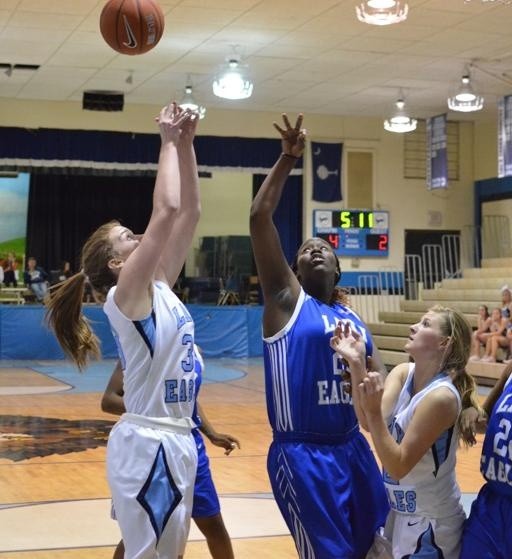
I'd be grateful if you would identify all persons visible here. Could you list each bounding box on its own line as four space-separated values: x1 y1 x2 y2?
0 251 20 289
25 257 48 299
54 261 72 285
42 99 201 559
249 111 391 559
471 287 512 364
101 329 243 559
459 357 512 559
330 303 486 559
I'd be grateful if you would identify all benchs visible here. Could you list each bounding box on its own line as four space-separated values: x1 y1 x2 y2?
364 256 512 386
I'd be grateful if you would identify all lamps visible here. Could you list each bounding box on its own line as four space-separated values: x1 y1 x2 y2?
382 67 485 133
175 85 205 120
355 1 409 26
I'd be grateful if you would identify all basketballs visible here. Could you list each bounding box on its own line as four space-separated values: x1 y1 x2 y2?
99 0 164 55
